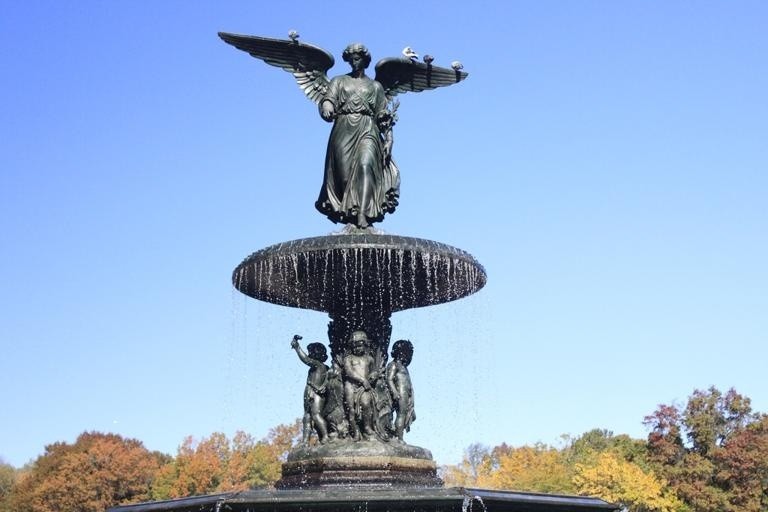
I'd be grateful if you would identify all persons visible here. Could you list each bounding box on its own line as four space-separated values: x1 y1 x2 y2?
341 329 378 442
315 44 400 236
386 338 415 446
290 338 328 446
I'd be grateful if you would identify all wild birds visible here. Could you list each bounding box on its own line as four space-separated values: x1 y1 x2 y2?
451 61 463 71
402 46 419 60
289 30 299 40
423 55 434 64
